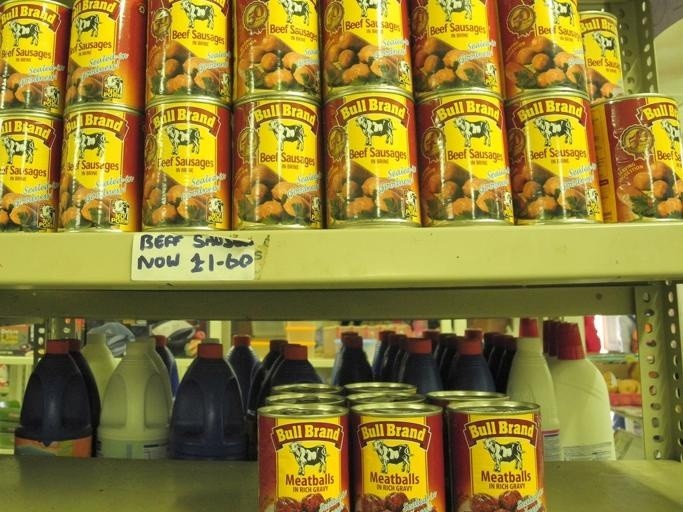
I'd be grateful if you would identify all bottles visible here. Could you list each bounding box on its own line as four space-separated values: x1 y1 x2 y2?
226 334 260 389
15 339 101 459
100 336 177 461
168 343 249 460
81 333 118 391
250 339 324 460
337 320 617 461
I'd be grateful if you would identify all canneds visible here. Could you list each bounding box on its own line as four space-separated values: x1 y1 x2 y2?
0 0 683 233
258 382 546 512
